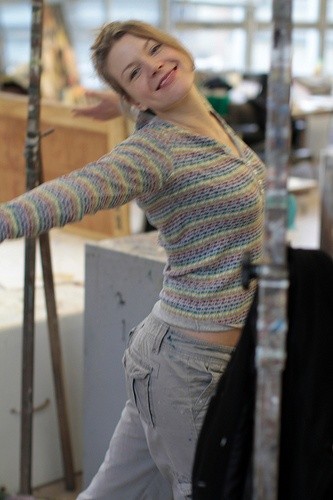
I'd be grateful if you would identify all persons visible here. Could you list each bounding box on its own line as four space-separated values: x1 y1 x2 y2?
1 20 268 500
